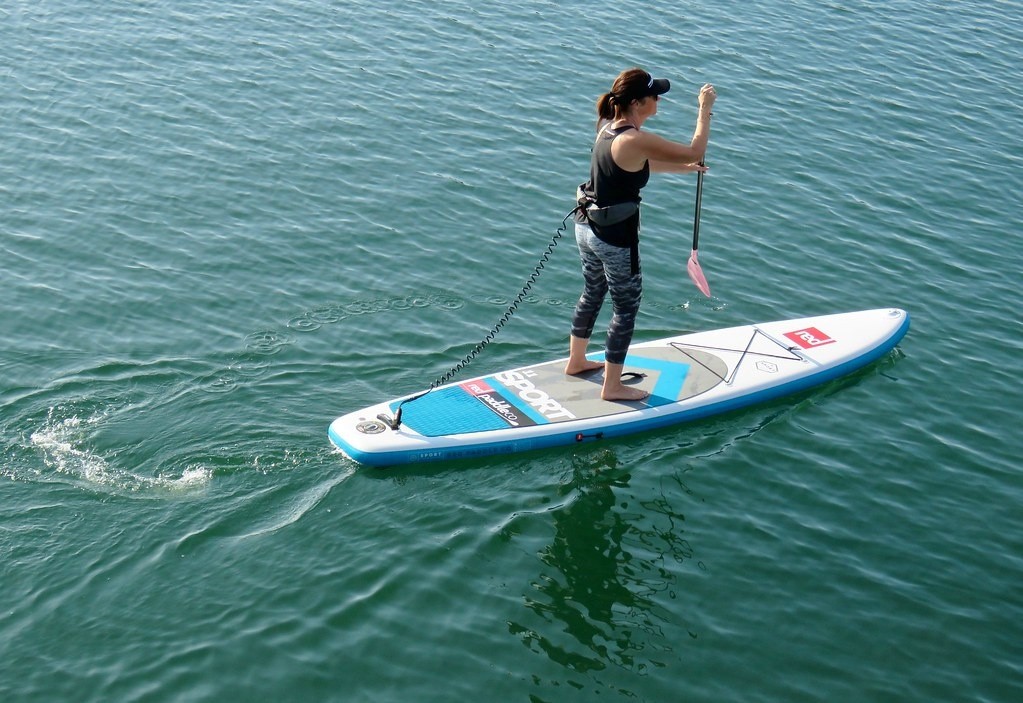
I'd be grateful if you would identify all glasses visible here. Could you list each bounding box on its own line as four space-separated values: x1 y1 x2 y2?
647 94 658 101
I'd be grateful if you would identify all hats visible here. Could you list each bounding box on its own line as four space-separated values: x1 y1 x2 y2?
615 71 670 105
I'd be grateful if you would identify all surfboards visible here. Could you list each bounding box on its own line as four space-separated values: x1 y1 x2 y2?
329 308 909 467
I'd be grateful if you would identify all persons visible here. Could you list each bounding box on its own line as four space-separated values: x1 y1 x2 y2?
565 68 717 401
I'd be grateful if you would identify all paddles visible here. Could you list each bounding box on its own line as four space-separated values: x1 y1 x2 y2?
686 99 714 297
